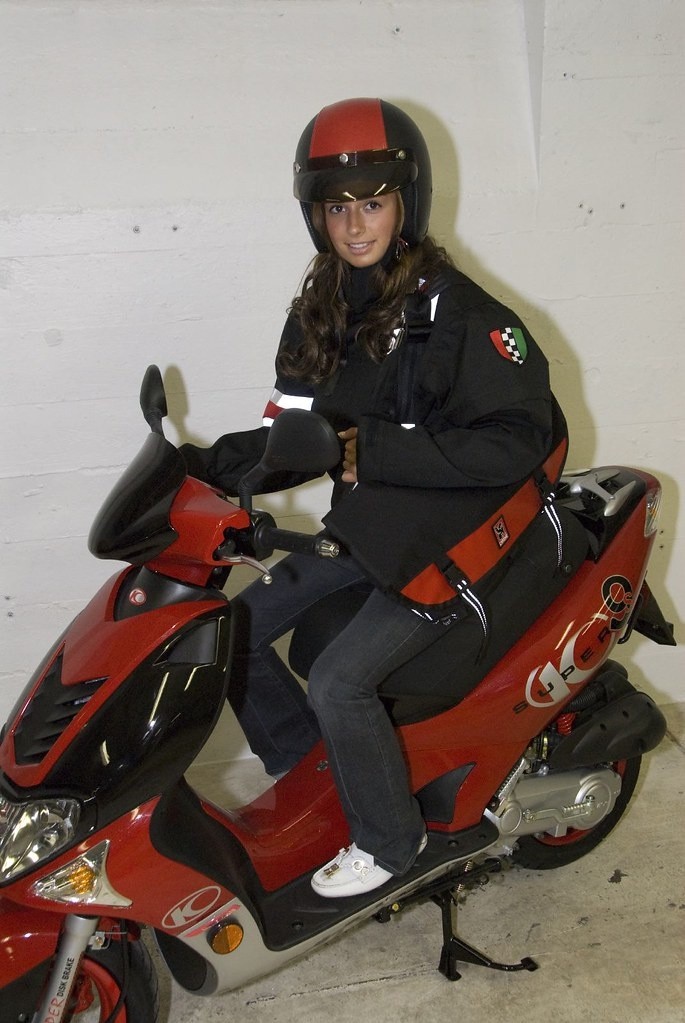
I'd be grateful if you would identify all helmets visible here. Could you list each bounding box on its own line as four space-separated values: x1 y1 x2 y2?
293 97 432 254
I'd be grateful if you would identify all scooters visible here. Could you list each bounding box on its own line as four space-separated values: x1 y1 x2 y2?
0 360 680 1022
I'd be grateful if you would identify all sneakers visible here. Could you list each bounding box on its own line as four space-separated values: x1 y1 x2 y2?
311 833 427 897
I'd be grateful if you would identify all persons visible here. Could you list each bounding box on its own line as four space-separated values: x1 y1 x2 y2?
177 98 554 897
